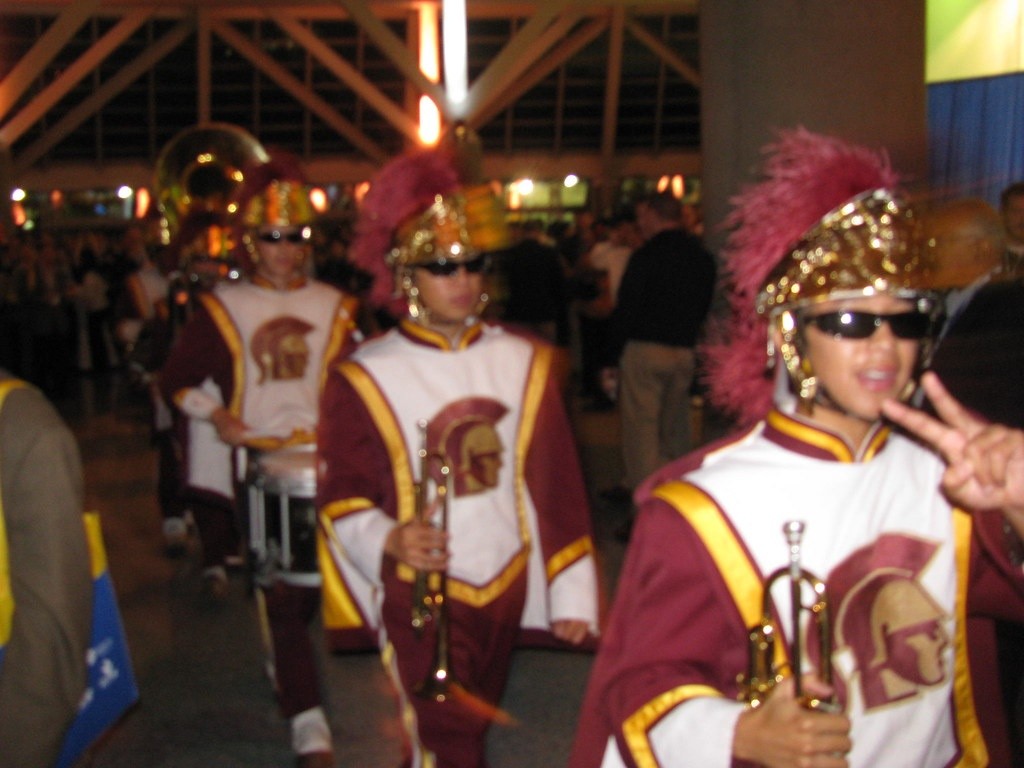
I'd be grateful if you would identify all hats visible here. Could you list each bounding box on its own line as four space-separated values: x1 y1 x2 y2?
174 214 243 261
241 152 326 230
702 128 1004 420
349 122 513 306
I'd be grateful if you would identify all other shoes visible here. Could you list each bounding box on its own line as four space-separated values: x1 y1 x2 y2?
164 518 187 554
616 516 634 542
291 706 332 755
225 556 248 577
202 565 227 598
599 485 632 504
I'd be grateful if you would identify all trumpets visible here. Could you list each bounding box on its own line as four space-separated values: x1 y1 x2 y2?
741 517 852 768
407 420 470 704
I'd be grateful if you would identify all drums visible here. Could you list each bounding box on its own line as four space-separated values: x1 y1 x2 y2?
247 445 327 589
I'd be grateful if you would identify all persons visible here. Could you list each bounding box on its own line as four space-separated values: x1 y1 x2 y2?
600 188 716 543
0 125 1023 768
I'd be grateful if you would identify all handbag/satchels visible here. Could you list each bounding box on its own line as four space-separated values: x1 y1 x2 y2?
0 376 139 768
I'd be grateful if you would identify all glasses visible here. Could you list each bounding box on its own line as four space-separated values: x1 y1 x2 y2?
409 252 492 274
257 231 307 245
797 311 933 340
